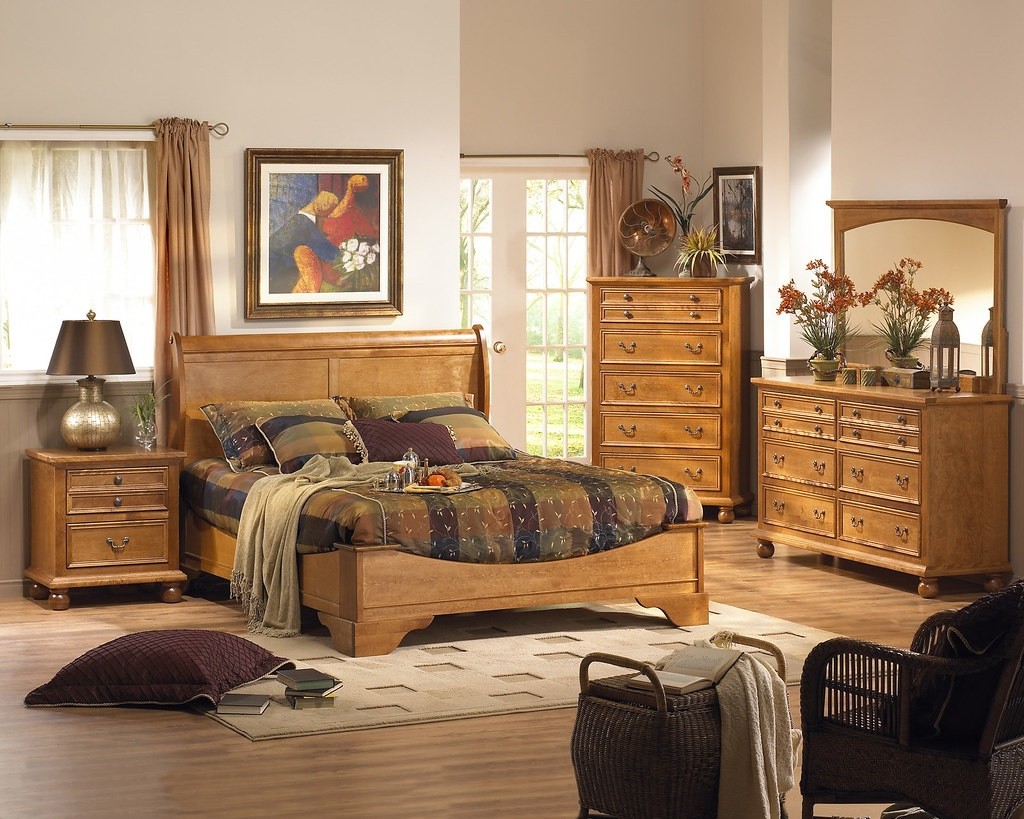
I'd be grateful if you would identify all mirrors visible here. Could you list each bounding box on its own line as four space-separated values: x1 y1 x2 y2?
825 198 1012 406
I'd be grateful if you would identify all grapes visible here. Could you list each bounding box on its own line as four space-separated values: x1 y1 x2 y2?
418 471 439 486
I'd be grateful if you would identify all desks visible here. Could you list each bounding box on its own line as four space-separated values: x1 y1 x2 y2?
570 631 787 819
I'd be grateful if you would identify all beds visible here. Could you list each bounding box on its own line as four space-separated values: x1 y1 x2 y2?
169 332 711 658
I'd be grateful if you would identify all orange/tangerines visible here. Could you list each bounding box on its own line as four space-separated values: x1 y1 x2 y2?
428 475 446 487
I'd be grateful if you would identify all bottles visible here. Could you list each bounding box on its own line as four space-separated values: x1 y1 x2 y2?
402 448 419 462
385 463 423 491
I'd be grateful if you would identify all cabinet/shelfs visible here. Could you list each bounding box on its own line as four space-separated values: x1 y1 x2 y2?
585 276 755 524
749 376 1013 599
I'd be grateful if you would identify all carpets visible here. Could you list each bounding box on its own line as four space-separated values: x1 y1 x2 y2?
205 597 921 742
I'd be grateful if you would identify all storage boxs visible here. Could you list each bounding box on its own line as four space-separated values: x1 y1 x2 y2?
882 369 931 389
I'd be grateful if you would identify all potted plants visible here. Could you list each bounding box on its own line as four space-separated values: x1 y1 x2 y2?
123 380 173 450
671 217 733 278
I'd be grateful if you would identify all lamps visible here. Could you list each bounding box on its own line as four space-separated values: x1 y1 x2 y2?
45 309 136 453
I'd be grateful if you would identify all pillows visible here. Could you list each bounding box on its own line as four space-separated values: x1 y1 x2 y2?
349 391 474 418
255 414 362 473
198 395 347 474
23 630 296 715
390 406 519 465
342 418 464 467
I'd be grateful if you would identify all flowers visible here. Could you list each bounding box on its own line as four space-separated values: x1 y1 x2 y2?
648 154 712 231
775 258 863 351
864 256 954 356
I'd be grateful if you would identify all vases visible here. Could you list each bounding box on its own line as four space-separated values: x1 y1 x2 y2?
809 352 840 380
889 355 919 368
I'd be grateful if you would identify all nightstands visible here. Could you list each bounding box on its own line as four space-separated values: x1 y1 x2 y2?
25 449 188 609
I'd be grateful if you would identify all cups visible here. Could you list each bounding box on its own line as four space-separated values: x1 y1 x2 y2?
842 368 857 384
860 370 877 386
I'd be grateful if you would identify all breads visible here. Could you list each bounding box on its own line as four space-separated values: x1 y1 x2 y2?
436 469 463 486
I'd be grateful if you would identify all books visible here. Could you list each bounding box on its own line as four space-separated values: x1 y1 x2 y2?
274 667 344 711
215 693 272 716
624 646 745 696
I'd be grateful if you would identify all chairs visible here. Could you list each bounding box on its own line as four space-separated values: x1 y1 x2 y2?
798 610 1024 819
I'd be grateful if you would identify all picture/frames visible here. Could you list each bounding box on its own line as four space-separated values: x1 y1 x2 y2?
246 147 406 320
712 165 761 265
